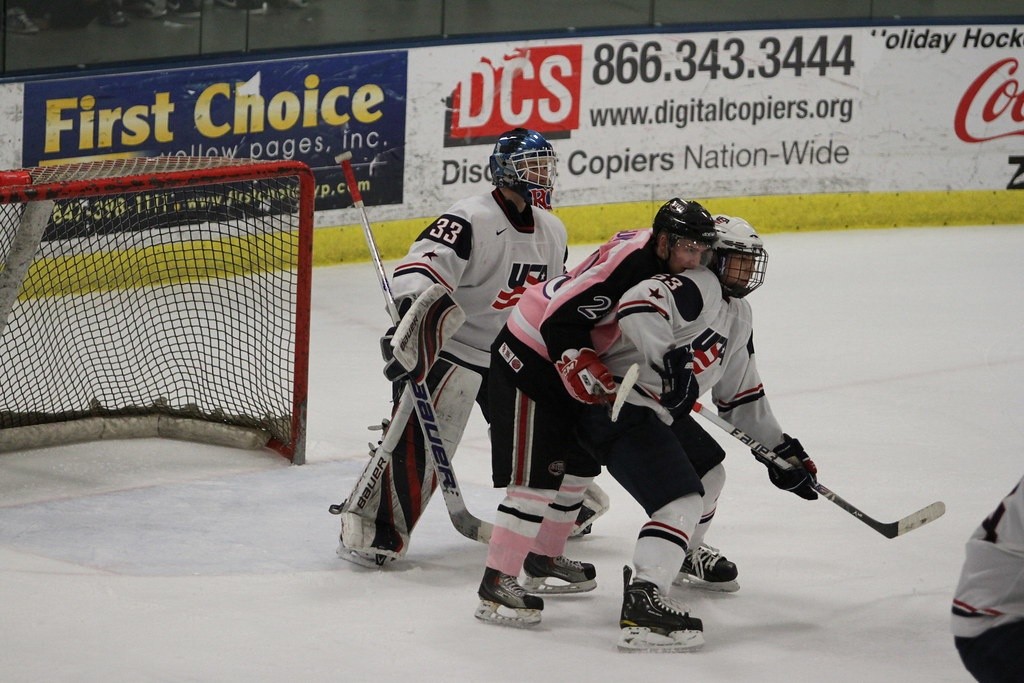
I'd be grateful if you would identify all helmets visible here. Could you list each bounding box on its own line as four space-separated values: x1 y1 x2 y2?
711 213 768 298
490 126 559 209
652 198 719 248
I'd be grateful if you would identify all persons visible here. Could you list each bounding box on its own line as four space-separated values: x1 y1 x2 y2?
329 127 609 569
475 199 720 629
950 476 1024 683
598 214 817 653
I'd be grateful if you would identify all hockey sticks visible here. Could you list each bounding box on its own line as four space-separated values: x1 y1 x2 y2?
331 149 496 545
593 362 641 424
663 385 948 540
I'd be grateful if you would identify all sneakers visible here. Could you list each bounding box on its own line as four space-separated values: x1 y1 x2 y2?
475 567 544 626
616 565 704 649
672 546 740 592
522 551 598 593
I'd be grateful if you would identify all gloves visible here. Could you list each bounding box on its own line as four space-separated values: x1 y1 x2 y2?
553 346 618 404
660 344 699 419
752 432 817 499
